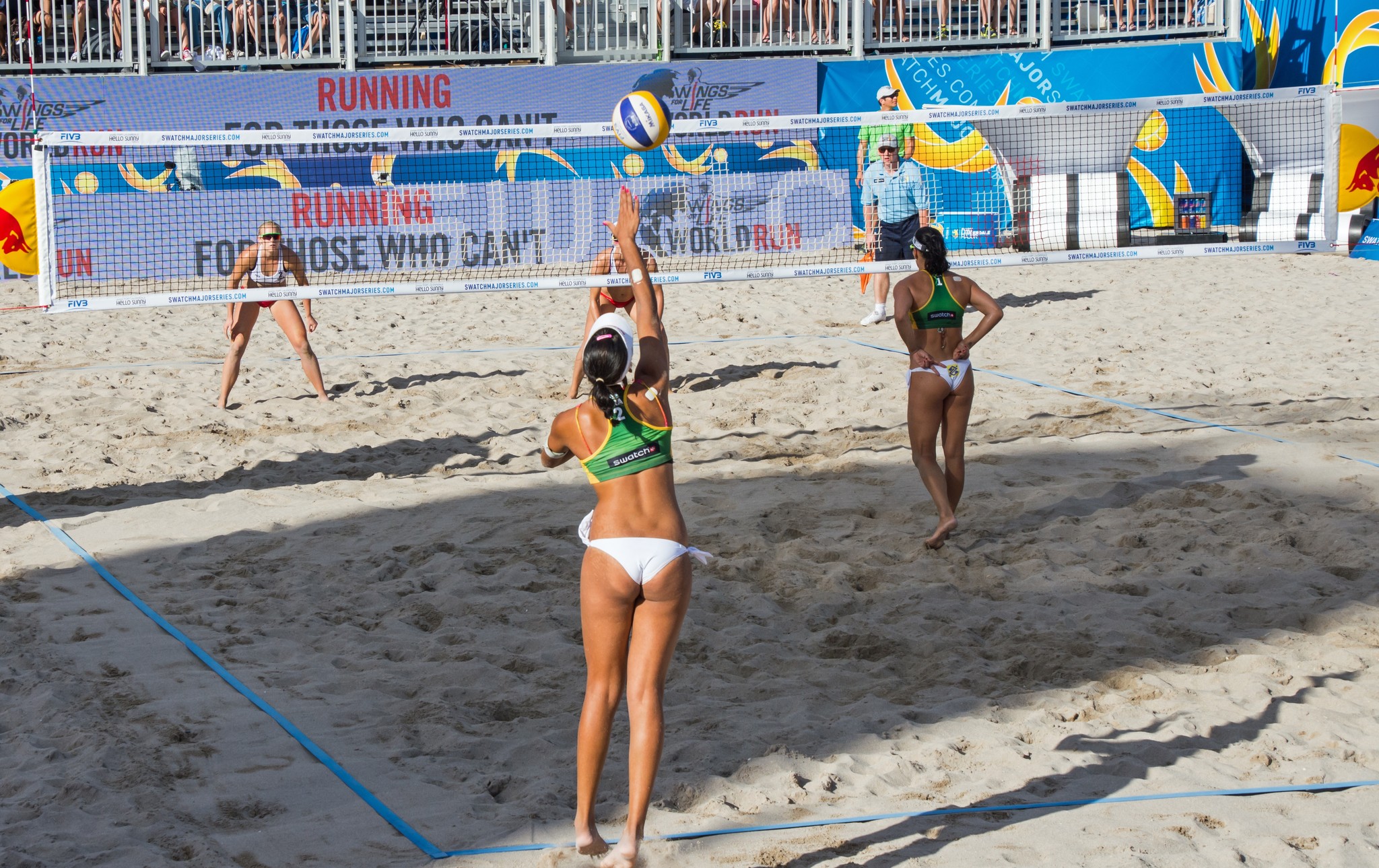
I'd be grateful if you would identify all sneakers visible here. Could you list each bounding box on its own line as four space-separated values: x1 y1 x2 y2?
70 51 81 60
565 25 583 49
115 50 123 59
981 23 997 37
936 24 949 39
160 47 193 62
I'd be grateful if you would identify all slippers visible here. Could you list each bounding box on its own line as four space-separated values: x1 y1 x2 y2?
762 29 795 44
811 37 836 45
1182 20 1203 26
1119 25 1136 31
1145 22 1156 28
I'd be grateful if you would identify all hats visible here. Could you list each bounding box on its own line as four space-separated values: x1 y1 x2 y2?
908 226 933 252
876 85 900 100
582 313 633 386
877 133 898 147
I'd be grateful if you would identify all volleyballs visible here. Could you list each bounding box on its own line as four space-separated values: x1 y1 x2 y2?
612 90 672 152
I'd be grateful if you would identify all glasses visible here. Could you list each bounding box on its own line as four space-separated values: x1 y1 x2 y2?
878 147 895 153
881 93 899 99
910 244 929 252
258 233 281 240
611 233 619 242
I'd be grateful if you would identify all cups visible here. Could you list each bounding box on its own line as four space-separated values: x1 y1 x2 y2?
61 5 72 19
869 50 880 55
752 23 759 31
147 65 155 74
121 67 133 75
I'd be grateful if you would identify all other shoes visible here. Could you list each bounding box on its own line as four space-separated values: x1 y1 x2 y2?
713 19 727 34
684 39 707 46
702 17 716 34
281 50 311 71
231 46 245 57
255 50 266 56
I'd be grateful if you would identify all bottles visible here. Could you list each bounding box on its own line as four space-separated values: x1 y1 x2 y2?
37 36 43 55
175 51 197 58
236 64 261 72
655 39 662 61
670 34 674 46
1163 13 1170 26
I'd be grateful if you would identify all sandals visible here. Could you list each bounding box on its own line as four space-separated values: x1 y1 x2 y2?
0 54 7 61
1009 26 1017 35
992 26 1002 36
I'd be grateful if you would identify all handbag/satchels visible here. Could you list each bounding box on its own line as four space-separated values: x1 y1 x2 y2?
291 24 316 56
1074 2 1112 31
81 27 117 60
451 20 490 51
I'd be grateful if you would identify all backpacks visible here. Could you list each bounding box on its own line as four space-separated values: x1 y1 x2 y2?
708 28 741 60
237 29 267 57
5 16 43 64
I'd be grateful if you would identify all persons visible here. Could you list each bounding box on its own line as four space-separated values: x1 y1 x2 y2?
892 227 1004 549
855 85 915 233
0 0 1203 62
217 221 330 409
860 134 929 326
564 222 675 399
542 186 693 868
